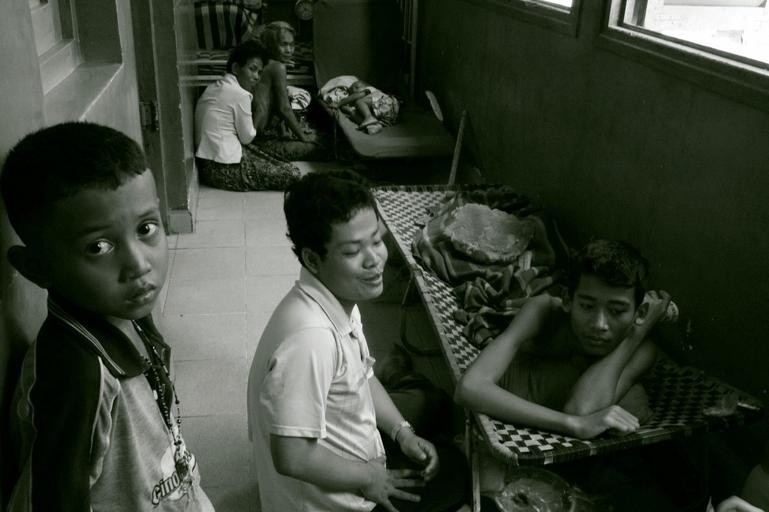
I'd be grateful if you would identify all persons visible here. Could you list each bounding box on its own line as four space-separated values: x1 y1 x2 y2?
452 228 673 441
248 163 472 510
329 80 399 135
192 37 301 191
1 118 214 512
252 20 335 162
715 495 769 512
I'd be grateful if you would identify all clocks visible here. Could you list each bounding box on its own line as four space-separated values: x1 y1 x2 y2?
294 0 313 20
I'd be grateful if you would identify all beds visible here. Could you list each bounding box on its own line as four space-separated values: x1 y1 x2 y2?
370 185 767 468
197 41 313 87
318 94 455 179
195 0 268 49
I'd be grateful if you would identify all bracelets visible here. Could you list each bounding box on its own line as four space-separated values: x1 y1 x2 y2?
391 421 415 444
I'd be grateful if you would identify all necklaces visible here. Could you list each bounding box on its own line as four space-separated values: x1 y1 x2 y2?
122 319 191 480
